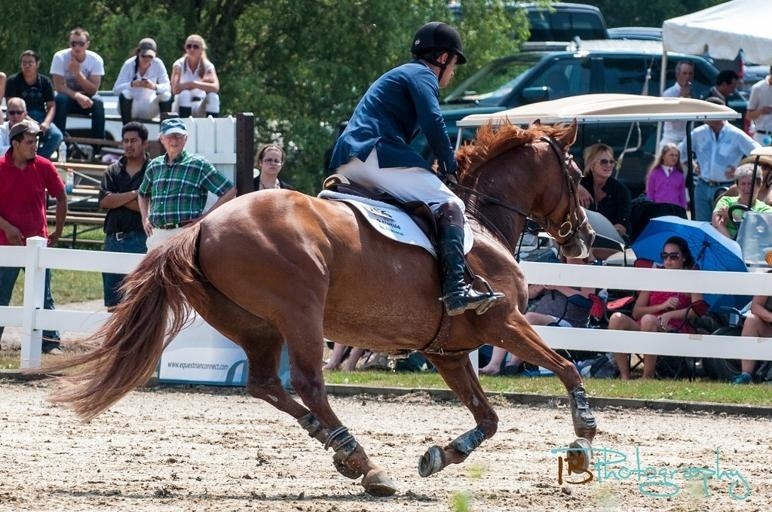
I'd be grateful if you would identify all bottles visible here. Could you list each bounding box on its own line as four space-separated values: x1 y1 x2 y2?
63 167 75 195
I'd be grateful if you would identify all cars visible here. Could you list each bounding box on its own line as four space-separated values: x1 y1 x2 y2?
506 2 662 42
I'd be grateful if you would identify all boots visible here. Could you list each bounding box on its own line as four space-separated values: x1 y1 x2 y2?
119 94 132 124
159 100 173 114
434 201 506 316
181 106 218 118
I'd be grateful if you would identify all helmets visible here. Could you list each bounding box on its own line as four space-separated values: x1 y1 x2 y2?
411 22 467 65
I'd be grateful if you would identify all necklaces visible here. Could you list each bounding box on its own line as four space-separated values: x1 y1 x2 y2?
260 180 278 189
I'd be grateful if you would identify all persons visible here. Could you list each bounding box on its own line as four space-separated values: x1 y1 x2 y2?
171 34 220 118
253 144 299 194
1 120 68 355
140 118 237 257
0 70 7 124
100 123 151 313
328 22 506 318
111 36 172 124
0 97 40 157
323 59 770 385
6 47 64 159
48 27 105 161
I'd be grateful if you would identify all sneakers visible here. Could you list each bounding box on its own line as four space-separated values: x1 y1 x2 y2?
732 372 752 386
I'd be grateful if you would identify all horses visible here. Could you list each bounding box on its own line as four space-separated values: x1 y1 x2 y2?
18 118 596 496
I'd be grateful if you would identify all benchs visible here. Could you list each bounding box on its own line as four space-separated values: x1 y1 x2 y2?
0 107 166 248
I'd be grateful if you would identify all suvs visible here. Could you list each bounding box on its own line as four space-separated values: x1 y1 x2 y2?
412 49 749 199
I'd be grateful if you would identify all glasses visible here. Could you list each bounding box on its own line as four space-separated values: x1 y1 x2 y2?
262 157 282 164
8 110 24 114
24 61 35 66
71 40 85 46
597 159 615 165
661 250 680 261
186 44 199 48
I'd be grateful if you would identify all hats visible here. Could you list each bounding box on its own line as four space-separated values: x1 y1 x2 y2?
139 38 157 57
8 121 44 137
160 118 188 137
740 155 772 167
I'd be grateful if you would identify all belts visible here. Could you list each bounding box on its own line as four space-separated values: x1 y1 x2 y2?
700 175 734 186
113 233 123 241
757 129 772 134
157 222 193 228
